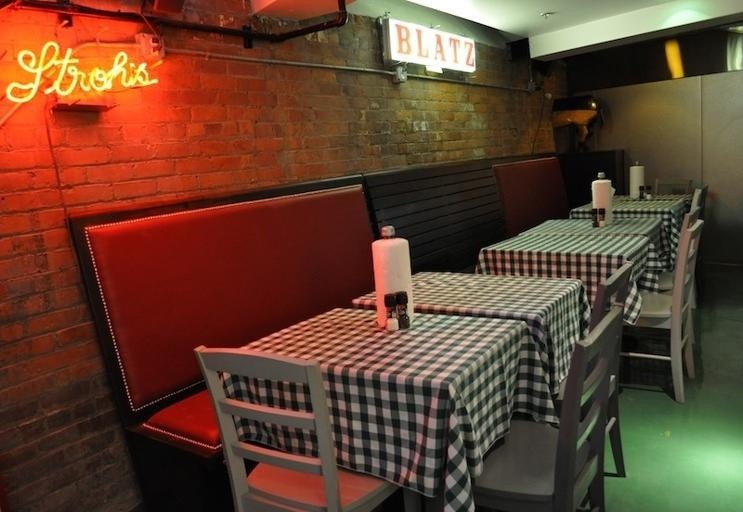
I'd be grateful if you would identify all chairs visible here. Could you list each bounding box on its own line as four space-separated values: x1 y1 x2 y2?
475 303 628 511
622 218 705 403
551 254 635 508
657 209 703 310
692 181 709 211
194 341 423 510
656 175 694 195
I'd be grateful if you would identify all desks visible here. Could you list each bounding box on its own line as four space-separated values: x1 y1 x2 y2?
214 299 563 512
352 268 595 424
475 213 663 327
568 191 693 267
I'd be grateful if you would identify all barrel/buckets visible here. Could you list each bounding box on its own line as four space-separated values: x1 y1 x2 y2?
591 178 616 225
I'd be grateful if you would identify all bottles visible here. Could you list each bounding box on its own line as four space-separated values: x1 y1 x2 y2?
591 172 614 227
371 226 415 332
629 160 652 200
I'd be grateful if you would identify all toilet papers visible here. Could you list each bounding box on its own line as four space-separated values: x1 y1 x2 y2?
372 237 414 326
629 165 645 200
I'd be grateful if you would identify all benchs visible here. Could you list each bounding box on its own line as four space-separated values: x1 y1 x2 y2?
68 174 389 511
493 152 569 233
